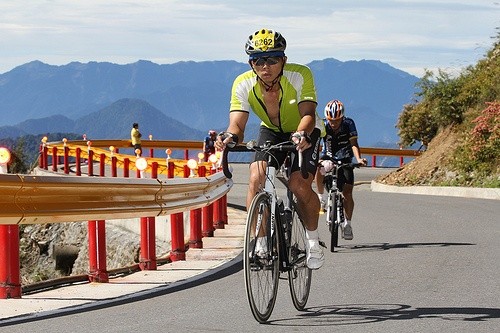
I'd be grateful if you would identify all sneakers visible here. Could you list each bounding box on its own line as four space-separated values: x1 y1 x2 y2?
249 246 268 259
306 243 325 269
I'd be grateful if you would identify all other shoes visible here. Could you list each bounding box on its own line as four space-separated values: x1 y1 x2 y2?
344 224 353 240
319 199 326 211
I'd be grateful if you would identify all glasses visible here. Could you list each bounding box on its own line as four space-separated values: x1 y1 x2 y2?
252 57 280 66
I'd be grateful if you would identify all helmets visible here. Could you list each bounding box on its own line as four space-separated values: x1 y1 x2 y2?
244 28 287 59
324 99 344 120
208 130 216 135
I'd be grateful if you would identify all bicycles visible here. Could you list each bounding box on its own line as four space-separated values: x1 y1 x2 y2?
219 134 328 325
318 159 369 253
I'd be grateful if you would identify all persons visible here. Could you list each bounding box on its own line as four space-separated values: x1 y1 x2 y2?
318 100 368 240
131 123 142 157
203 131 218 162
213 28 327 270
